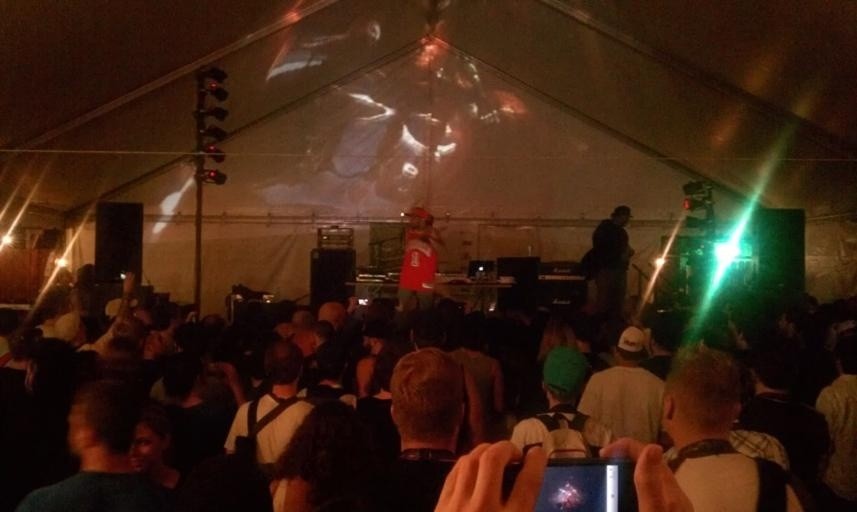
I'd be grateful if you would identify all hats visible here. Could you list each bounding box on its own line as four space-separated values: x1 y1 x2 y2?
103 298 137 318
610 205 633 217
542 345 589 396
54 311 80 342
404 207 429 220
609 323 649 362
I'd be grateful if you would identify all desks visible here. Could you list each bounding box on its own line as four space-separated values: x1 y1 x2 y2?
342 280 513 312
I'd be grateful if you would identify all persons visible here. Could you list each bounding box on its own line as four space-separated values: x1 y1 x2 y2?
590 204 638 312
1 257 856 510
399 203 444 309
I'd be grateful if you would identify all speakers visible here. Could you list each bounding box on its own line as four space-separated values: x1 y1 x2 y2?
94 201 142 282
310 245 356 305
496 256 540 314
754 207 804 288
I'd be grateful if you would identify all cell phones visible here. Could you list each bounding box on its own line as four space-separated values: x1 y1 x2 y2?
503 458 638 512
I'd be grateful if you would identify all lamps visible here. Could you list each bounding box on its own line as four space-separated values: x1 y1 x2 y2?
682 181 717 266
193 68 231 187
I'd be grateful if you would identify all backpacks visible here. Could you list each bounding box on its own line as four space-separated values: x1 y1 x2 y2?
533 411 593 460
226 395 298 511
